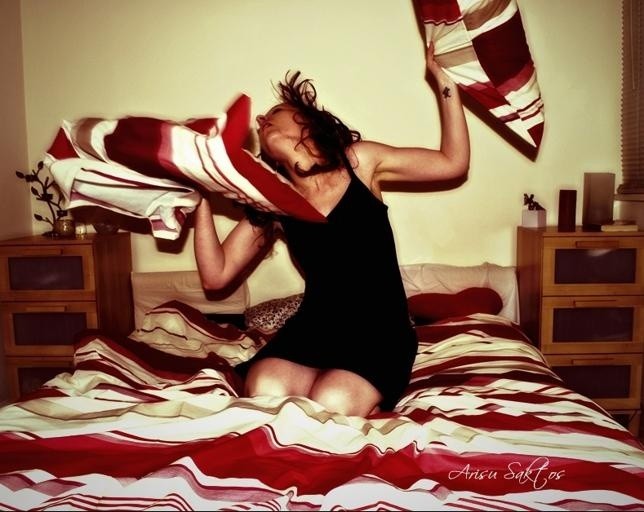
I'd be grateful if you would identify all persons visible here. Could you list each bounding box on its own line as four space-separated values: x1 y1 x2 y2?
193 39 472 420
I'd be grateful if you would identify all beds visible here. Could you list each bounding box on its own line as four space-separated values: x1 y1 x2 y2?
0 321 644 510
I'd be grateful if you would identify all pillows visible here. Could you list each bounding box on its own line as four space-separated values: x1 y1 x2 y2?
400 262 523 329
413 0 547 154
127 270 252 329
40 93 324 245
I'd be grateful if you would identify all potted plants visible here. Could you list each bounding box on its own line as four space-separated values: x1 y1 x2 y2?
521 193 547 228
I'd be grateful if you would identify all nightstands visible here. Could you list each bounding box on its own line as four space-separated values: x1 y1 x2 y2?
0 231 135 403
515 225 643 428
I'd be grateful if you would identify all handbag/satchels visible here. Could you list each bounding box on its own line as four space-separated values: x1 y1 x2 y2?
244 293 305 335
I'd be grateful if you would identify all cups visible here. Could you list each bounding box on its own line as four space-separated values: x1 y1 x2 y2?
558 188 577 233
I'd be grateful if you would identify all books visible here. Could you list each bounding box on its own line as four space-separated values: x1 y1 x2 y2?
600 219 639 232
